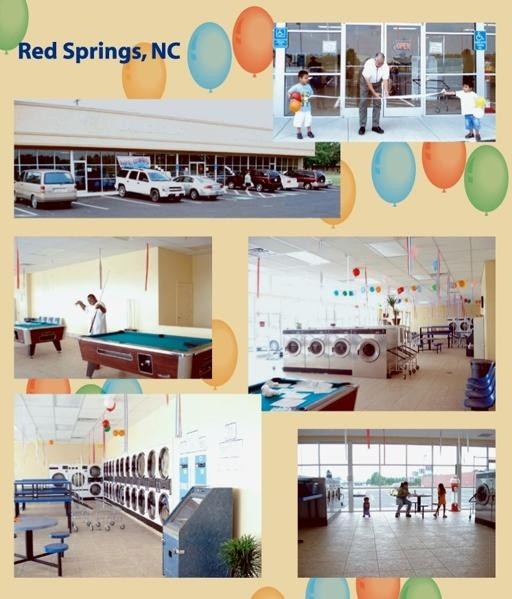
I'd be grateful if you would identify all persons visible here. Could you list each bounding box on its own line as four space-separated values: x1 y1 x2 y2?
285 54 292 68
76 296 107 335
287 71 318 139
307 57 324 90
432 483 447 519
358 54 391 135
442 84 486 142
395 481 412 517
362 497 371 518
243 171 252 193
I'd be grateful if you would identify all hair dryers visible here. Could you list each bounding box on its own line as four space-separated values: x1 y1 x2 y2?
298 478 341 526
104 443 174 527
283 330 305 372
305 330 329 373
353 326 397 379
447 317 473 333
329 330 352 375
475 470 495 530
49 464 104 499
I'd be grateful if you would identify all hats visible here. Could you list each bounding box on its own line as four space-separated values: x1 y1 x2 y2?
172 175 225 201
321 171 333 188
278 174 297 192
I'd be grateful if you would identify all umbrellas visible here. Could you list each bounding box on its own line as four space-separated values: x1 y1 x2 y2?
297 133 303 139
372 126 384 133
476 134 480 140
308 131 314 138
465 133 474 138
359 127 366 135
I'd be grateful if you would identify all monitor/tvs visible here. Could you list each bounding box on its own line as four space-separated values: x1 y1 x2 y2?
252 576 442 599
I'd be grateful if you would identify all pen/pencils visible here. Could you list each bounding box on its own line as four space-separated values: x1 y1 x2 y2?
14 168 79 210
225 169 282 193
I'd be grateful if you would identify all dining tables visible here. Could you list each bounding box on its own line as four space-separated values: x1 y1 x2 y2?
393 310 400 324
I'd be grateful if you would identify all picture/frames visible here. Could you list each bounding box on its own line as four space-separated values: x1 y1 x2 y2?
14 321 65 356
78 330 212 379
248 377 359 411
14 515 61 568
14 478 72 533
409 494 432 512
419 326 453 352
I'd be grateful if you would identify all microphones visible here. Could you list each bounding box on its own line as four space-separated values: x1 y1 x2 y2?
410 502 418 513
419 505 428 519
433 343 442 353
44 543 68 576
48 532 70 558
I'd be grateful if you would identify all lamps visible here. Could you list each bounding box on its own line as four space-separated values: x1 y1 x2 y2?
465 362 495 408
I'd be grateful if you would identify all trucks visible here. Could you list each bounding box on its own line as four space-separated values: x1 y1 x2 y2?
122 6 276 99
326 261 478 322
45 439 55 447
475 97 486 108
325 141 508 232
289 90 302 113
27 378 145 395
98 397 129 438
0 0 28 55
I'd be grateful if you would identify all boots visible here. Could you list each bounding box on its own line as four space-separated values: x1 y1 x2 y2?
113 167 186 203
293 169 326 190
